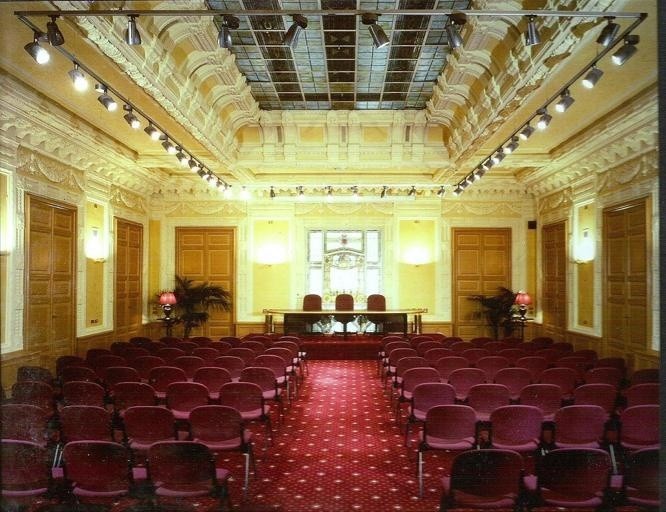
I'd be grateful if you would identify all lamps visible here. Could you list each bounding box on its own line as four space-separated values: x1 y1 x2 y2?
217 15 240 48
281 15 308 46
23 38 233 198
436 34 640 197
515 289 531 320
593 17 621 47
444 13 467 47
47 16 64 46
160 291 177 320
361 14 390 48
126 16 141 46
270 188 276 198
525 15 542 47
408 186 416 196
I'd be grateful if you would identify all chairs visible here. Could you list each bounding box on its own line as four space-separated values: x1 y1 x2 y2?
363 295 388 337
334 294 355 339
301 294 324 336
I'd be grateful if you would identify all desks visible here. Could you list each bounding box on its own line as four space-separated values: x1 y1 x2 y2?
512 317 535 341
263 309 427 332
156 318 179 336
